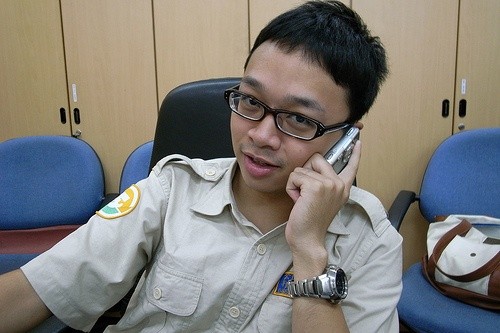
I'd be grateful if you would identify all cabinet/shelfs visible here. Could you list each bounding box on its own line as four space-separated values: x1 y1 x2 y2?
152 0 352 112
0 0 159 194
350 0 500 274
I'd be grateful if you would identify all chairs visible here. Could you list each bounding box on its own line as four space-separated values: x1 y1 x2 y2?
0 134 106 333
384 126 500 333
54 76 243 333
97 140 155 212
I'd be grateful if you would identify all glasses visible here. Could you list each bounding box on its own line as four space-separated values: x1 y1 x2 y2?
225 84 351 141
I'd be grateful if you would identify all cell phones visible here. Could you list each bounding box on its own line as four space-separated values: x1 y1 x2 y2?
323 127 361 175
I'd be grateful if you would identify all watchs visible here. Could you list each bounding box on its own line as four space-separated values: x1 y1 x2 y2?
288 264 348 304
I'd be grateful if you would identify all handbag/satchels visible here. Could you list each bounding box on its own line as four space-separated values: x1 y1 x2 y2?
425 214 500 312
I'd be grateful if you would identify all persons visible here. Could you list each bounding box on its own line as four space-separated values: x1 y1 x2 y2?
0 0 403 333
